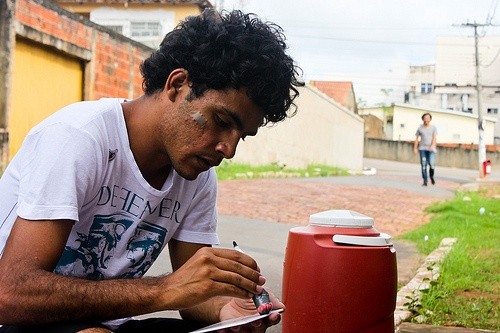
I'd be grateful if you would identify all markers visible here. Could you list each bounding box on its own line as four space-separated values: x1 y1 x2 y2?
232 241 271 316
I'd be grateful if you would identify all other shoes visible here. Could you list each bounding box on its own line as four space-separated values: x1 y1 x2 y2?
430 177 435 184
422 180 427 186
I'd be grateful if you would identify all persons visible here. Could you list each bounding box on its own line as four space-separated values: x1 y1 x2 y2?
1 6 306 333
414 112 437 186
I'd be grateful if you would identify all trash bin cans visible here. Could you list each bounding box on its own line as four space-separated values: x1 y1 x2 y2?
281 209 397 333
483 159 491 175
